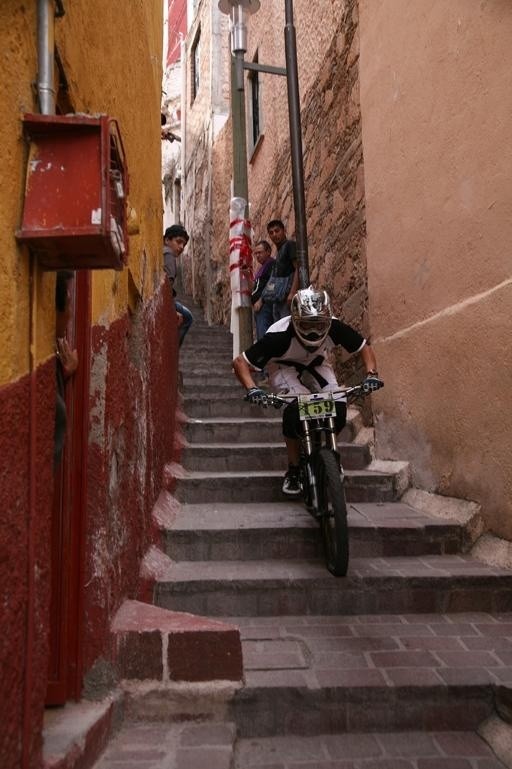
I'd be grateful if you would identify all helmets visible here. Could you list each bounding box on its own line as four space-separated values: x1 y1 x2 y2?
290 283 335 352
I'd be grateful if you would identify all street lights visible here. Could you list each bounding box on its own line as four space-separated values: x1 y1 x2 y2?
217 1 263 357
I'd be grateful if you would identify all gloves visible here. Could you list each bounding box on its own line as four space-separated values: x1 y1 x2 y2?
246 386 269 410
362 368 384 394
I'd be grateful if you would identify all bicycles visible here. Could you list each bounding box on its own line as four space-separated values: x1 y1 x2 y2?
243 378 381 578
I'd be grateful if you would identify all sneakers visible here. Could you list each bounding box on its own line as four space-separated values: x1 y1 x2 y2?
281 461 307 496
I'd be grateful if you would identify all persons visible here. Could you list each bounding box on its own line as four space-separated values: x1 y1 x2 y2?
231 285 381 500
54 270 81 483
266 218 299 321
252 239 275 372
161 224 195 355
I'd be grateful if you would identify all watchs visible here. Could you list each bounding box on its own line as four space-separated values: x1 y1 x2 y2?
367 369 378 377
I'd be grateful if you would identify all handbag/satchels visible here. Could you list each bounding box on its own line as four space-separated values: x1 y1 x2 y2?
260 275 293 306
